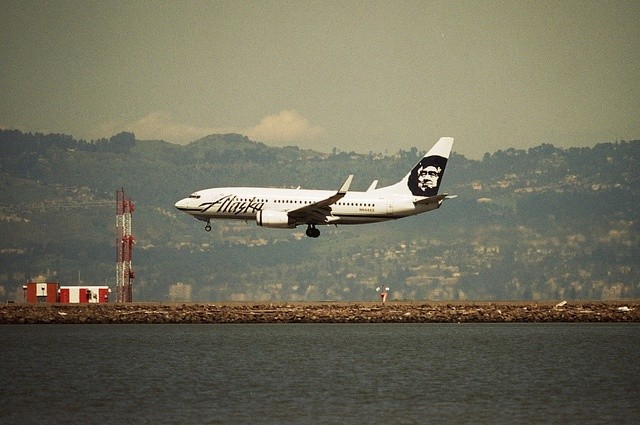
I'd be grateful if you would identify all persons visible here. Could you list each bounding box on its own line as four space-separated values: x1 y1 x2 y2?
416 165 442 192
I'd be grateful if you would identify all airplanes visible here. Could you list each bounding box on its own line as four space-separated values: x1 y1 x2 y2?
175 136 457 238
